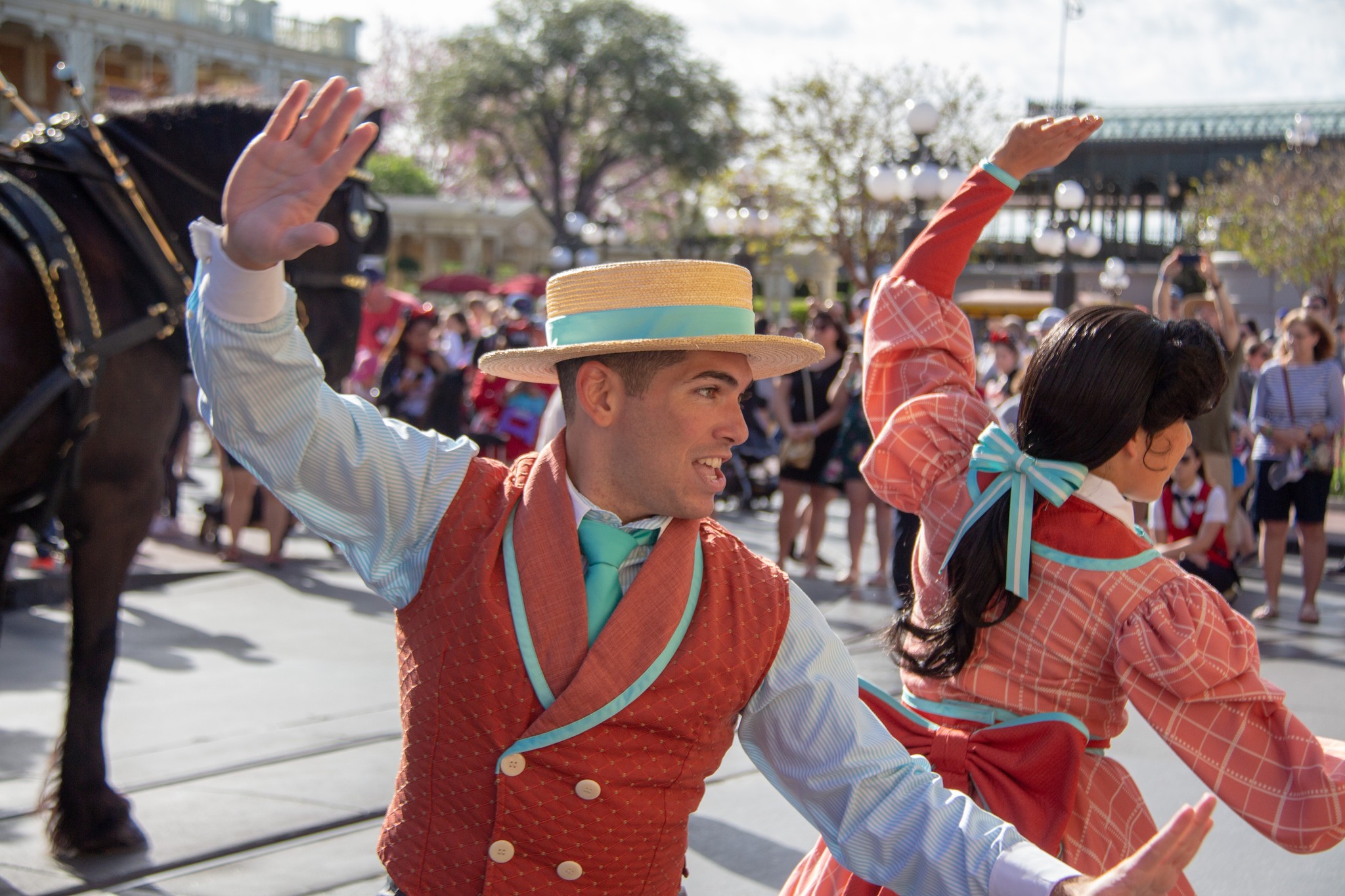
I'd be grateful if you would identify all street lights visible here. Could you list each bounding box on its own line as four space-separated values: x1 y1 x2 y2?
861 101 965 266
1034 182 1100 310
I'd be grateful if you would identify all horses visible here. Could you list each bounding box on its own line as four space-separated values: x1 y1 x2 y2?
1 85 396 871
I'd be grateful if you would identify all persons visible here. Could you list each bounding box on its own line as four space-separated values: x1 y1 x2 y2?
1148 445 1240 603
221 448 289 565
780 113 1345 896
977 309 1068 434
1230 294 1345 624
1152 246 1248 501
769 289 920 606
344 269 558 461
188 75 1219 896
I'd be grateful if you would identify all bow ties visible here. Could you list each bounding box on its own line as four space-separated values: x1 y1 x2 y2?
1172 494 1197 505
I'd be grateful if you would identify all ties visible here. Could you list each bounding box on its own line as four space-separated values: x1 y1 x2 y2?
578 515 660 649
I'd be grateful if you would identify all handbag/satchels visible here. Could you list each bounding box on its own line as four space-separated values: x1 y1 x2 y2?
1268 446 1304 490
780 436 814 469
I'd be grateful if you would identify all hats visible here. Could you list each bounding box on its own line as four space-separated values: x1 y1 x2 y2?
479 259 825 385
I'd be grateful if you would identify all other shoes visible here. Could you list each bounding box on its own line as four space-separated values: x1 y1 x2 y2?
1299 601 1318 624
1252 604 1278 620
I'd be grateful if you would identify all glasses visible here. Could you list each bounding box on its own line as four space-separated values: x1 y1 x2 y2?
1179 455 1190 464
